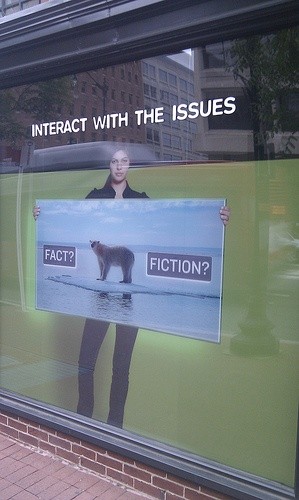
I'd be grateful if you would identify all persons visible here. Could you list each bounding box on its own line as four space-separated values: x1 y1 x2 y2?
32 146 230 429
